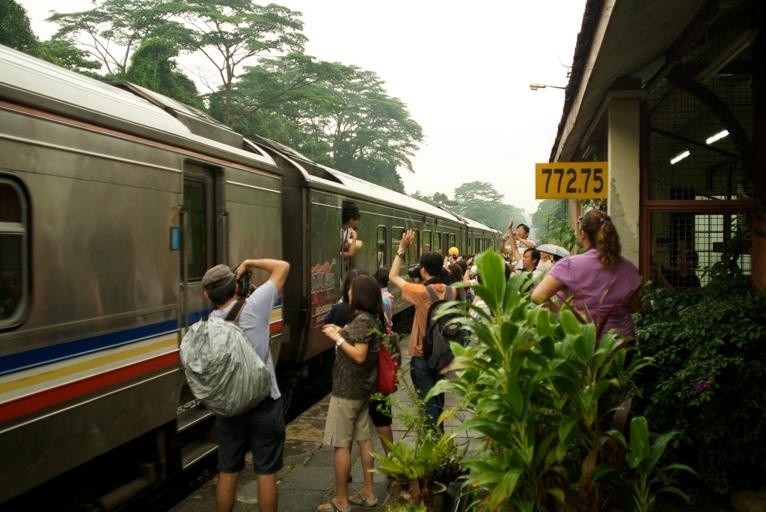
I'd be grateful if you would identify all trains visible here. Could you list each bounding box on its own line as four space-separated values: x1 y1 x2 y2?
0 42 505 512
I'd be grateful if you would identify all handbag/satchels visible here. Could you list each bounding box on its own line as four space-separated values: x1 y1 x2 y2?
377 326 400 394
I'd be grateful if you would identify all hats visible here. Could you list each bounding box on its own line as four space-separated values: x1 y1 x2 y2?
202 264 236 293
448 247 459 257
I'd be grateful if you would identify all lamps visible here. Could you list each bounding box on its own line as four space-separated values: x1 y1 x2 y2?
705 128 730 146
529 83 566 91
669 146 692 166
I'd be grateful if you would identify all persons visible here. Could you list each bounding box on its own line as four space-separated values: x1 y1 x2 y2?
462 208 642 477
654 246 700 296
201 257 290 511
388 229 469 435
319 206 403 511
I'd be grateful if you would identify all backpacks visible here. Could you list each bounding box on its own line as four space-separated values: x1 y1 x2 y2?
418 284 465 373
179 299 271 418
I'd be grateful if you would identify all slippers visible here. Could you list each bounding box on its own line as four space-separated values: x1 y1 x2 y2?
347 490 379 507
318 497 353 512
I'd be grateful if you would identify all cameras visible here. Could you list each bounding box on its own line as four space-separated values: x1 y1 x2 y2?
233 269 250 296
